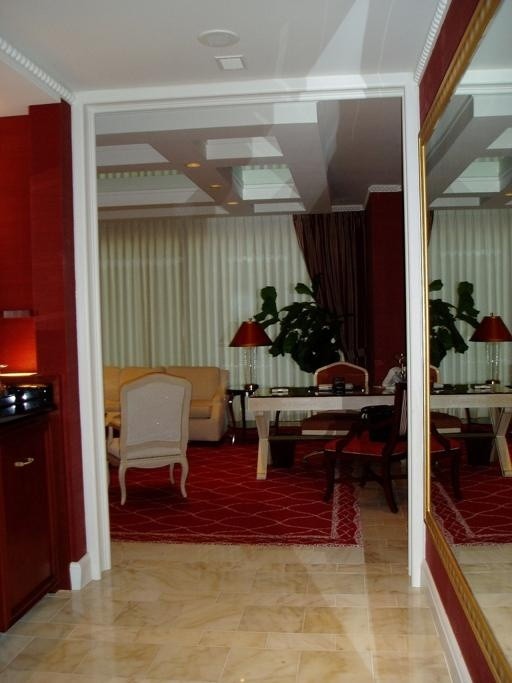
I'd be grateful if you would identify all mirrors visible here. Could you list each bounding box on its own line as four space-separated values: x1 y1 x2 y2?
417 0 512 683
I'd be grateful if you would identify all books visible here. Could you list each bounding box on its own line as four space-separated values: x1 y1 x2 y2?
270 387 308 397
470 381 492 390
318 381 333 390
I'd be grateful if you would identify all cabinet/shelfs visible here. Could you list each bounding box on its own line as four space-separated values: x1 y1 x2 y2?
0 401 63 632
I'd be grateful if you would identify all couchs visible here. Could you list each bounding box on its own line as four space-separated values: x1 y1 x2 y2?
104 367 228 446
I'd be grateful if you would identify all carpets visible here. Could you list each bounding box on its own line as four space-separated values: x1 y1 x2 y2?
107 426 362 548
431 438 512 546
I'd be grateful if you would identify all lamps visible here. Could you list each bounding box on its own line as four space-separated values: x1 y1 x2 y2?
468 313 512 384
229 318 273 390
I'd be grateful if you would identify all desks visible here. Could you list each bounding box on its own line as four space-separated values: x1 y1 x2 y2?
248 386 407 481
429 383 512 478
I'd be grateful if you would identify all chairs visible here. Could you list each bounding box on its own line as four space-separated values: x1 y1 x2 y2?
302 360 407 514
106 372 193 506
430 365 468 500
